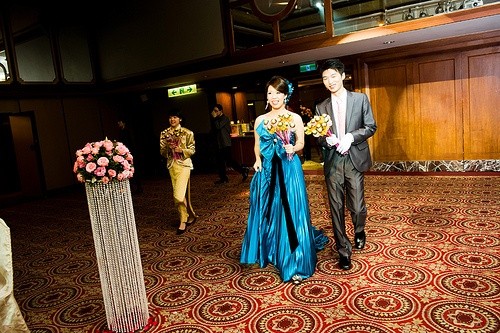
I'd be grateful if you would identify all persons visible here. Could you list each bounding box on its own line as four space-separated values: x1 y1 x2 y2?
211 104 250 183
239 75 328 284
316 59 377 270
160 109 199 234
116 116 143 193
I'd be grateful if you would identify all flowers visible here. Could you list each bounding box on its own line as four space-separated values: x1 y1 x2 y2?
160 126 188 161
263 111 297 162
303 113 349 156
73 136 134 188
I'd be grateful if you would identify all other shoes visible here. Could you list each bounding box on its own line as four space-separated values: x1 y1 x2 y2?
242 166 249 181
186 217 195 225
177 224 185 234
215 179 228 184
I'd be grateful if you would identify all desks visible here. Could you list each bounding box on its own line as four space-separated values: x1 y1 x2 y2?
231 134 257 166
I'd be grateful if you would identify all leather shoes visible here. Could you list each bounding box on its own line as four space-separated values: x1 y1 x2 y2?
355 230 365 249
340 254 350 270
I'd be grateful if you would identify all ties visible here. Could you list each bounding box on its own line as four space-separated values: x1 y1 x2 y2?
336 100 344 142
